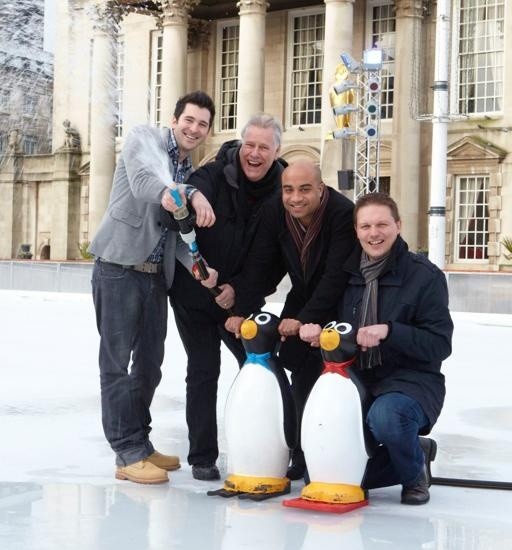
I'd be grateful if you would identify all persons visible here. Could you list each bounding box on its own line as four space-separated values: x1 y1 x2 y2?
159 111 291 482
86 89 216 486
223 155 360 480
297 191 455 503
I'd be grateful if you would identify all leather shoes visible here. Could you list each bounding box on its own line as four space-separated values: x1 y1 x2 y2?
401 436 436 505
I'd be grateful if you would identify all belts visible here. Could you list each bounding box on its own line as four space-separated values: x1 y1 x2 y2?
123 262 157 274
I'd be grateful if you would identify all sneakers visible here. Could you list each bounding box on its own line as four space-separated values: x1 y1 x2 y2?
115 449 179 485
193 463 221 480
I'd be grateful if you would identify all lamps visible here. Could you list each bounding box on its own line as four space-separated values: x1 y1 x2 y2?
332 52 381 144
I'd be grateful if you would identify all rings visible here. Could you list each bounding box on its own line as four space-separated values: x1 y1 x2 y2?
224 303 228 307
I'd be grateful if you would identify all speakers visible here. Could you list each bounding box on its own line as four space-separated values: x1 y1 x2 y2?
337 169 354 190
